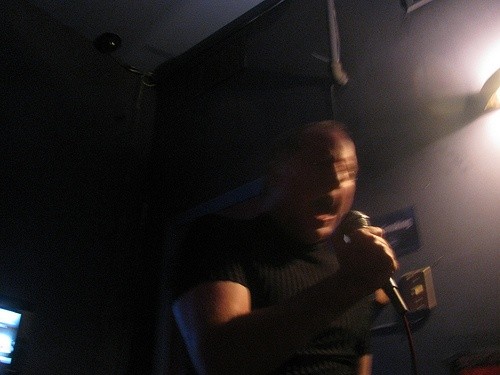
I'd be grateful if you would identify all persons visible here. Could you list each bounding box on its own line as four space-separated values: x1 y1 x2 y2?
165 119 397 375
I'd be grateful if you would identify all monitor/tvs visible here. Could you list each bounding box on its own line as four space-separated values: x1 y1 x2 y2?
0 305 32 370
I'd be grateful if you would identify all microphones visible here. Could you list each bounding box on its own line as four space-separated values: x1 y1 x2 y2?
341 211 409 315
